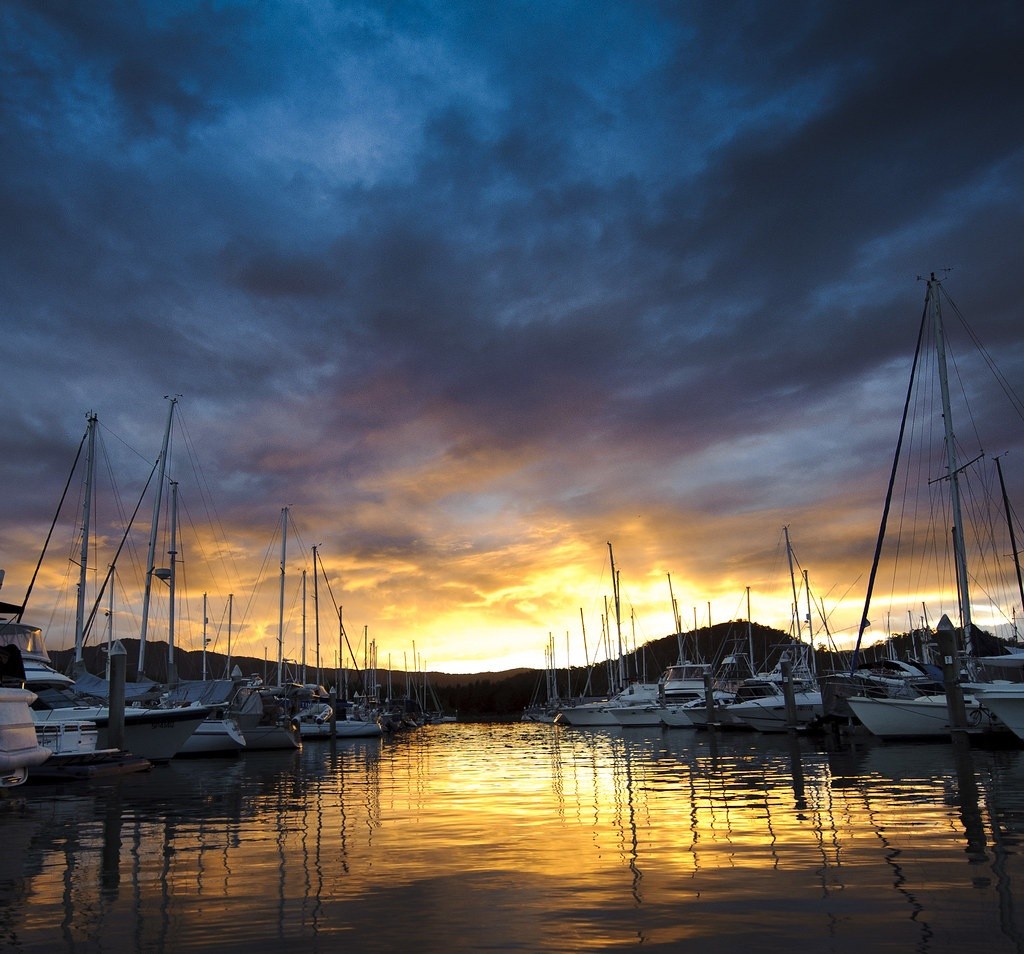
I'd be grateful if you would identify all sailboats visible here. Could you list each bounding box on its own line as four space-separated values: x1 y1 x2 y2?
519 272 1024 747
1 391 459 788
